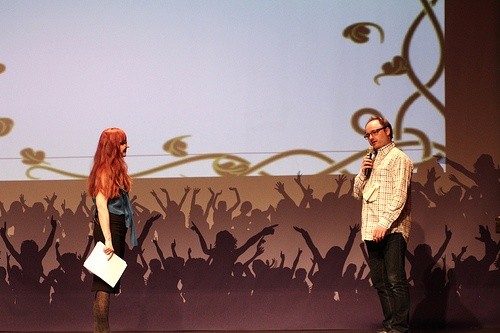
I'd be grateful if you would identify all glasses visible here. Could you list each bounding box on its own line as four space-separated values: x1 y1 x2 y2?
364 127 386 140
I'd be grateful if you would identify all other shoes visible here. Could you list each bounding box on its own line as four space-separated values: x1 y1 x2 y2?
377 327 400 333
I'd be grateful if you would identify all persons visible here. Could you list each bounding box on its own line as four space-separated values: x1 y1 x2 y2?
87 127 132 333
351 116 413 333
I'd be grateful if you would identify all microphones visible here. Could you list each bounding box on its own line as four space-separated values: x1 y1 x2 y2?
365 146 374 177
